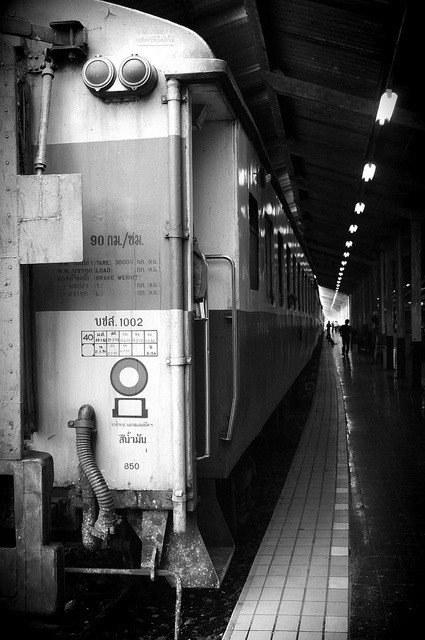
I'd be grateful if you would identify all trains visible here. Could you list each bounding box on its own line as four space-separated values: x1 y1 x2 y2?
0 0 324 640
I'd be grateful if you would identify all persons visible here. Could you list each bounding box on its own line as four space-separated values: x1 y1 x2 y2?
326 320 331 335
339 319 353 356
